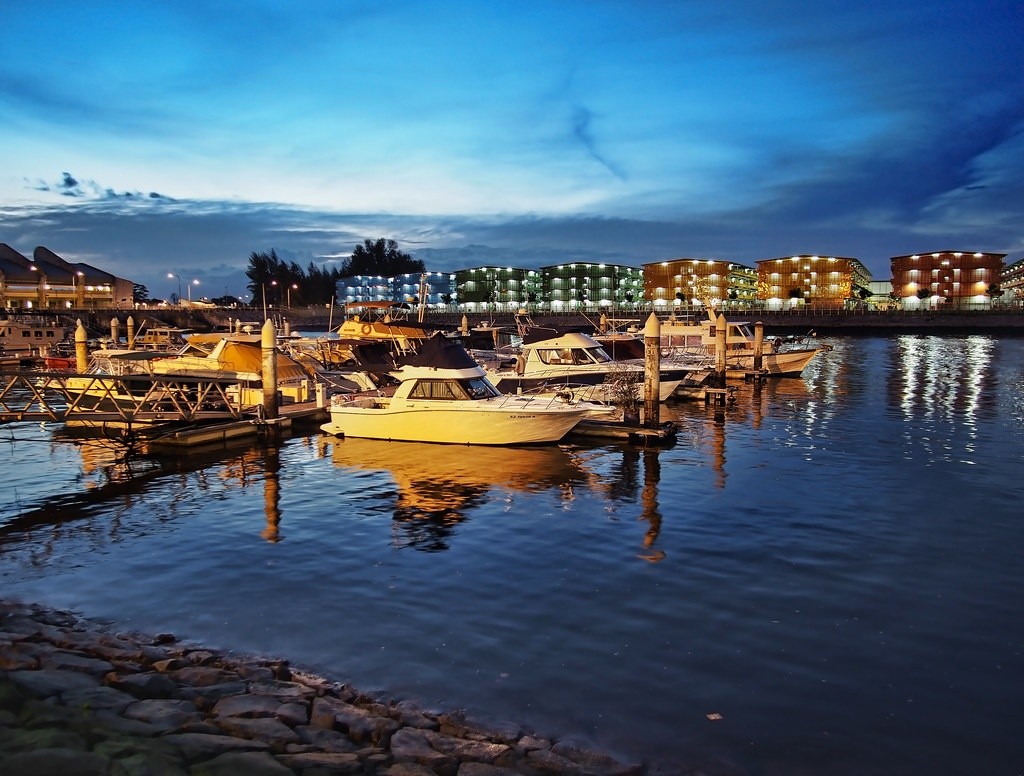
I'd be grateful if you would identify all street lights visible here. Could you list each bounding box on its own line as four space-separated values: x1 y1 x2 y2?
288 284 298 309
272 281 283 306
243 295 249 307
73 271 85 307
238 297 242 307
188 280 199 301
168 273 181 303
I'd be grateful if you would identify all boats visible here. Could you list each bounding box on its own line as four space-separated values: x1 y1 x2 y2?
431 297 819 402
0 314 333 424
726 376 811 401
319 328 592 444
337 274 429 339
319 436 587 512
